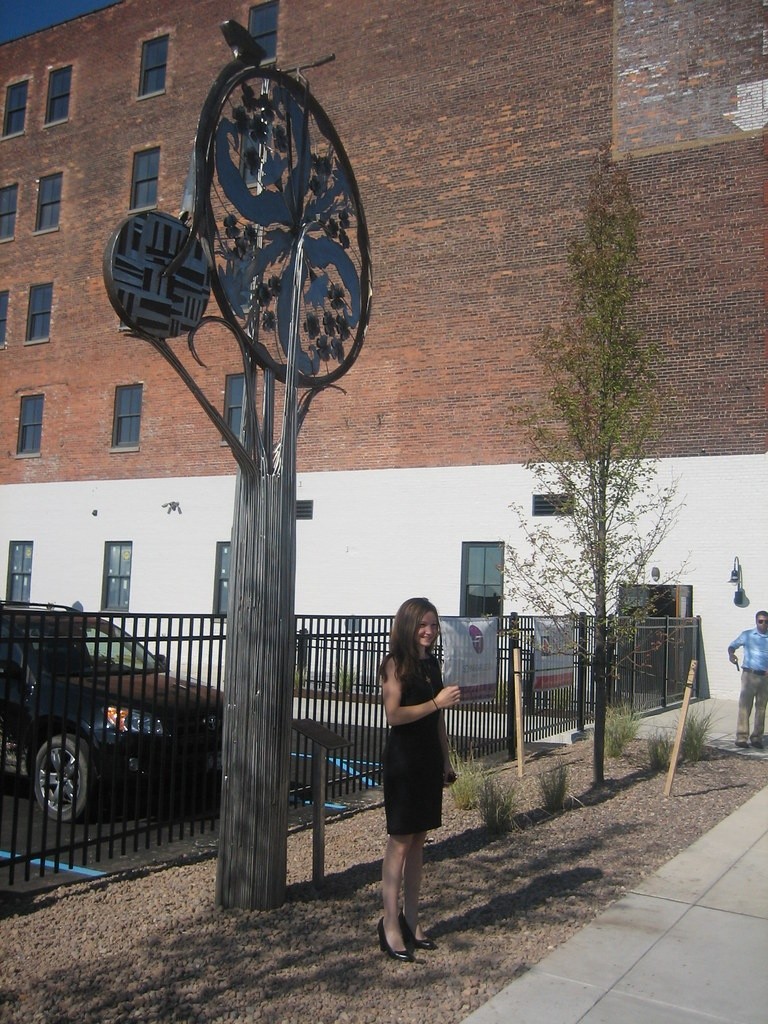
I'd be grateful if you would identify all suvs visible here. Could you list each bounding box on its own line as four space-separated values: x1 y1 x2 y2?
0 599 224 826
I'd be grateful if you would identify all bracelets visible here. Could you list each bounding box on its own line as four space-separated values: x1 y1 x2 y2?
432 699 438 710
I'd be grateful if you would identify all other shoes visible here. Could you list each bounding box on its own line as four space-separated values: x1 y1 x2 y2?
752 740 762 749
735 742 749 748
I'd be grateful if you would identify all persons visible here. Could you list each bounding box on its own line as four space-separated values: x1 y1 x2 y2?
728 611 768 749
377 598 460 962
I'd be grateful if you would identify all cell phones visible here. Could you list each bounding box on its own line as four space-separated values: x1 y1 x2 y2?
736 661 739 671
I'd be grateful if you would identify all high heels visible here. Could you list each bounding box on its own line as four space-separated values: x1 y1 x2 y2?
398 911 438 950
378 915 415 963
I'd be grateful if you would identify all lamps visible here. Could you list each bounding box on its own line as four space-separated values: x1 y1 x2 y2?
729 556 750 607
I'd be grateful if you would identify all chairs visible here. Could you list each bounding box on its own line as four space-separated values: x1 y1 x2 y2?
44 624 94 668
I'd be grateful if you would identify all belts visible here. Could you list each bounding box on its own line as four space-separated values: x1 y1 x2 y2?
743 668 766 675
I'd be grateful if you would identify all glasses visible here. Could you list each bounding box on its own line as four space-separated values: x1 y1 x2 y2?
757 620 768 624
